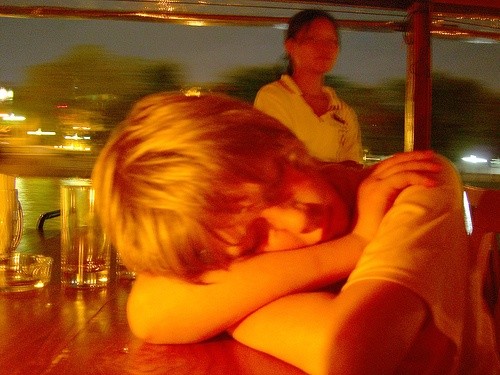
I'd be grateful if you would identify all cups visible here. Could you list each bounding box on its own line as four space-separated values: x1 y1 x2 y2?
60 180 111 290
0 173 23 261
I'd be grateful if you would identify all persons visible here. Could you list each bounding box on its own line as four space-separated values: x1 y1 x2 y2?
88 90 500 375
250 7 365 170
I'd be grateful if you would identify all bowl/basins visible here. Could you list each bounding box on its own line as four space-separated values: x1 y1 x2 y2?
0 252 55 293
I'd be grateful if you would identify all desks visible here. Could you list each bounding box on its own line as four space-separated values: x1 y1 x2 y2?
1 226 310 375
